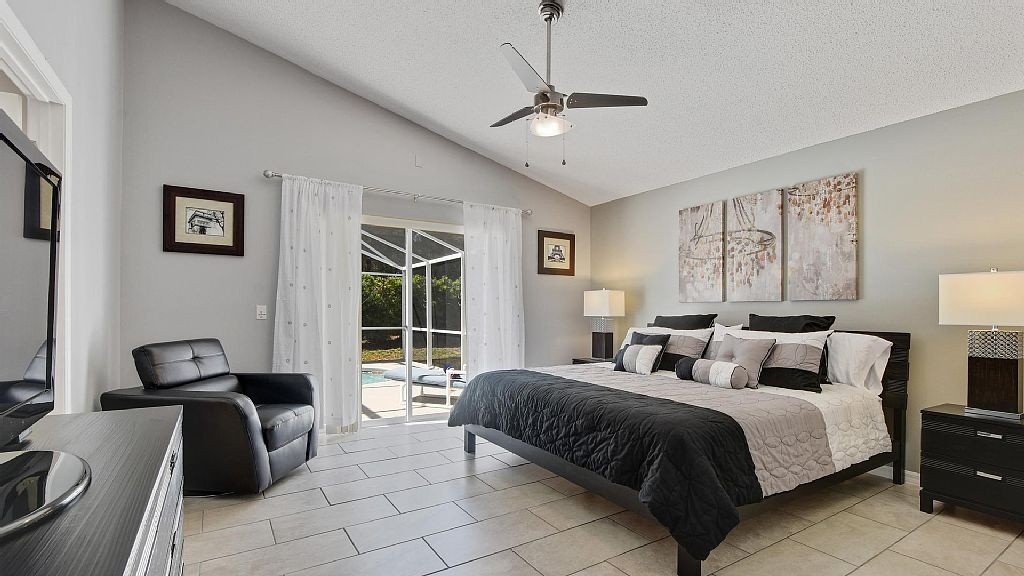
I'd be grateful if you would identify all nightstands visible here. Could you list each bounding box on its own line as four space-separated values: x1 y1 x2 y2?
919 403 1024 524
572 357 613 365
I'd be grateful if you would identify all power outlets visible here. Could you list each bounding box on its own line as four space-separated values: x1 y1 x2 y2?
256 305 270 319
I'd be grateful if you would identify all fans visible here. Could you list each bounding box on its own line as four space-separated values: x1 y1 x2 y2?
490 1 648 128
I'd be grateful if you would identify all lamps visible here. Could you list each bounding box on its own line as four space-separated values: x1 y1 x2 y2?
939 268 1024 419
524 85 572 168
584 287 625 359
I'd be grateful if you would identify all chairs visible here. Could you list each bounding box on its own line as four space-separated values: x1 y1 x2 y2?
383 367 466 409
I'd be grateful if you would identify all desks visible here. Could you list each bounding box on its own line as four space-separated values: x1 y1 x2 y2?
0 405 185 576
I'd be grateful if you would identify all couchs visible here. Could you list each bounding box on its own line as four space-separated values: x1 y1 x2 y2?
100 338 319 495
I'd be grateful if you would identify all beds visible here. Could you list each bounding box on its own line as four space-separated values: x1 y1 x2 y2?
447 330 911 576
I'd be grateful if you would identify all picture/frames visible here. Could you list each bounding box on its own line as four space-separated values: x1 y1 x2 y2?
162 184 244 256
538 230 575 276
23 162 53 240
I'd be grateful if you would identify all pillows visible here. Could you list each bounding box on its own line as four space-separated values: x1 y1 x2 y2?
612 313 893 396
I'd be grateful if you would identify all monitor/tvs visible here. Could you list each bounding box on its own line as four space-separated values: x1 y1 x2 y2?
0 107 93 541
